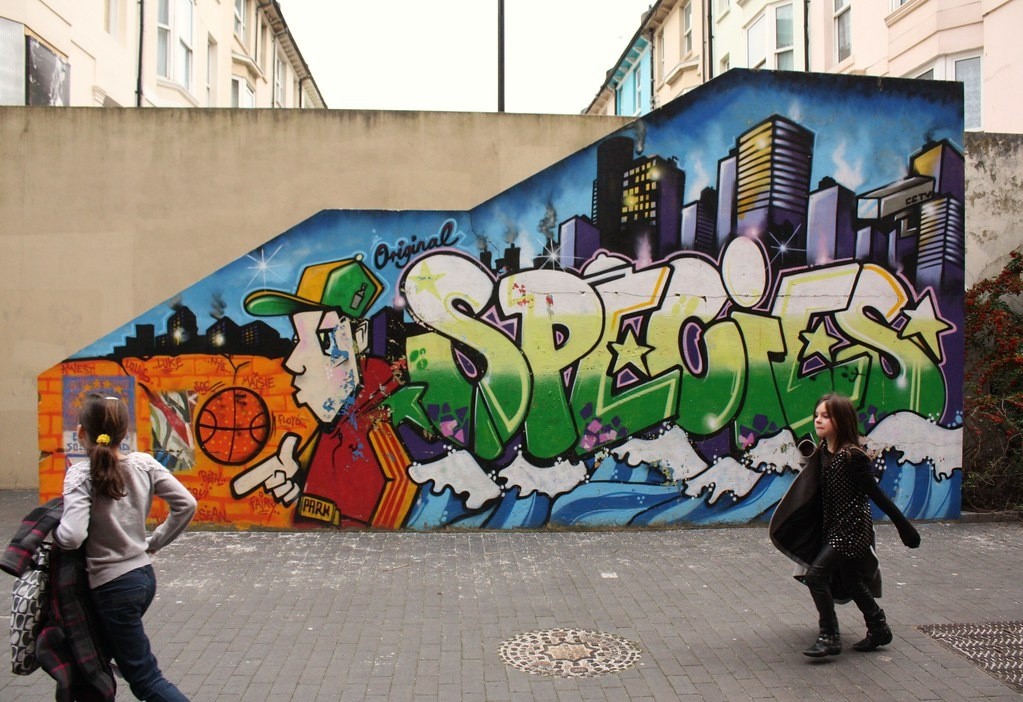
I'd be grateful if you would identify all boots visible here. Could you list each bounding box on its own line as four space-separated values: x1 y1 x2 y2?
801 617 842 658
851 609 894 651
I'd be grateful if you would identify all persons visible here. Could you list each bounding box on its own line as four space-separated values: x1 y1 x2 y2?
55 386 198 702
803 391 922 659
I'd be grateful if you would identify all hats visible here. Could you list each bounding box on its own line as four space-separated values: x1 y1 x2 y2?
244 254 385 324
